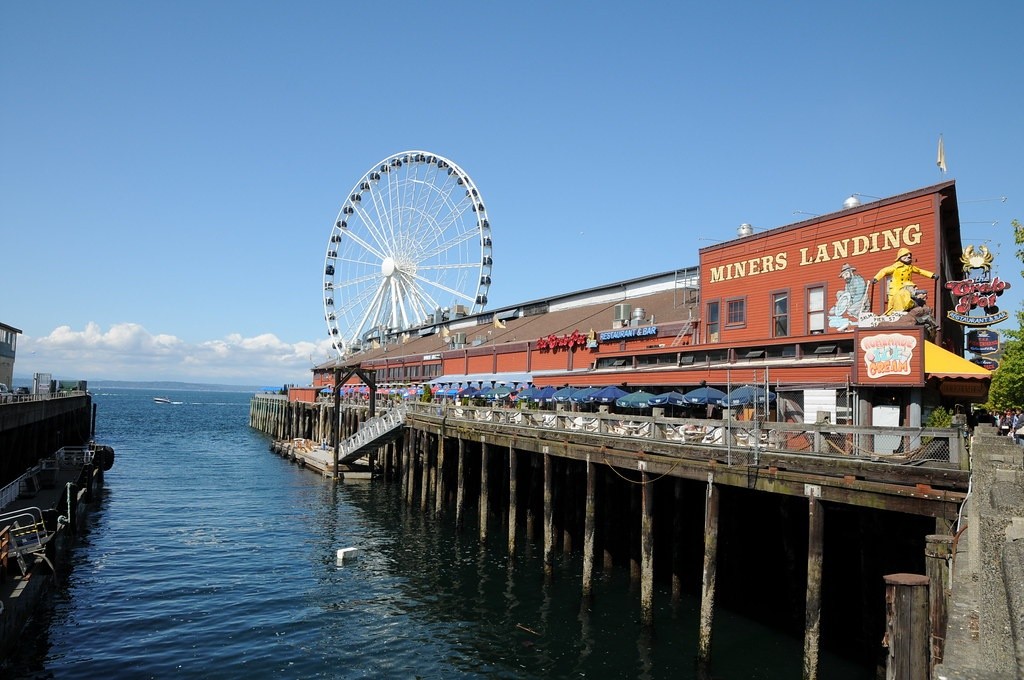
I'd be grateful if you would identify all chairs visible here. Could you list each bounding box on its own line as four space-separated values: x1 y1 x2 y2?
454 402 782 456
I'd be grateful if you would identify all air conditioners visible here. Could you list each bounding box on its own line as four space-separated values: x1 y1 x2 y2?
456 332 467 344
613 302 632 321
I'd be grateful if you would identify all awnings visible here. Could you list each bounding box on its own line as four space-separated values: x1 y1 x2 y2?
924 340 991 379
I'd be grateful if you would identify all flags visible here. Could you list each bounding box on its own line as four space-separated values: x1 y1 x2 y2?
373 341 380 349
345 348 352 355
402 334 409 345
937 136 946 171
442 326 449 337
493 317 506 328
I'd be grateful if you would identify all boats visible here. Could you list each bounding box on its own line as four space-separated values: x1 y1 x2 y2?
154 397 171 404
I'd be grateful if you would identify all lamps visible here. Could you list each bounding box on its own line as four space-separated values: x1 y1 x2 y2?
958 196 1008 275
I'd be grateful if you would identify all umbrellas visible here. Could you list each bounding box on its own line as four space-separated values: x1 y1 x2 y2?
516 385 558 410
648 391 684 417
552 385 580 411
587 385 629 414
569 386 601 413
615 390 656 416
683 386 727 419
721 384 775 420
456 385 516 407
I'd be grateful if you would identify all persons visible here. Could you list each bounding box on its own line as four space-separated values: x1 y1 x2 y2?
871 248 939 314
907 290 941 332
957 405 1024 446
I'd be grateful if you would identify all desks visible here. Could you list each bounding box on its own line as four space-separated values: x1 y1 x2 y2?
685 430 706 435
737 433 767 440
666 429 673 431
627 425 639 435
